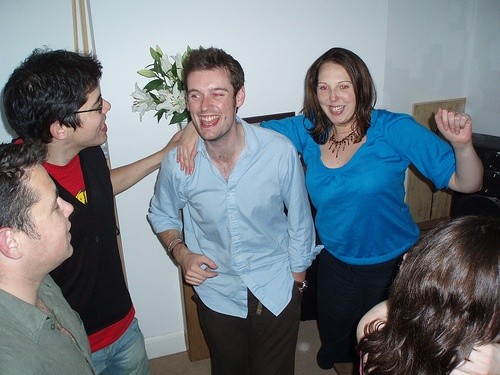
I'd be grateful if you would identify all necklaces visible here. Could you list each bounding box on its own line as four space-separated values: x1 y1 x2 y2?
329 126 356 159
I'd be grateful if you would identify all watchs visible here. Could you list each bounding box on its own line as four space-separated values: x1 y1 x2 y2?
292 279 308 292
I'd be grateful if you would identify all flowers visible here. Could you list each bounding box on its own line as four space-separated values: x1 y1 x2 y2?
129 43 191 131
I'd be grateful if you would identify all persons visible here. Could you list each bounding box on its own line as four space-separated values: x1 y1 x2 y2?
356 214 500 375
0 46 184 375
175 48 484 375
147 47 324 375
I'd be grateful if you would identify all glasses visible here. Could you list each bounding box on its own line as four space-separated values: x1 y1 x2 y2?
73 98 103 115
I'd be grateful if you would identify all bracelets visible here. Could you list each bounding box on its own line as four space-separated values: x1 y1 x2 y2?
169 239 185 259
